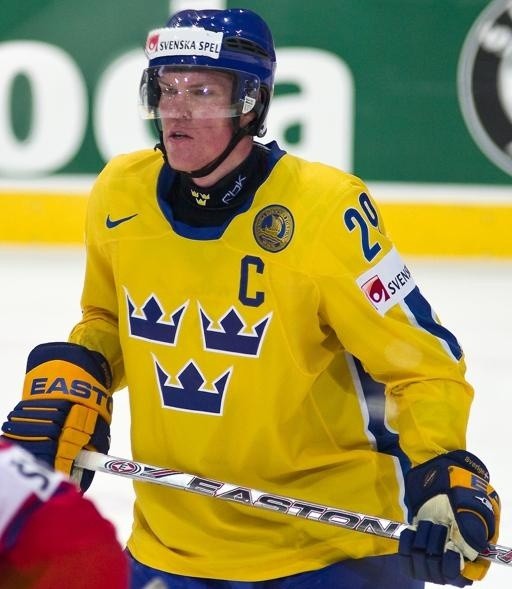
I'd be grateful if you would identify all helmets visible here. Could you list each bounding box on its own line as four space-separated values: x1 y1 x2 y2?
136 6 279 136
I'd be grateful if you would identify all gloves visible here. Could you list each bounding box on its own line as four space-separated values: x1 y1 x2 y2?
396 448 502 588
0 342 114 497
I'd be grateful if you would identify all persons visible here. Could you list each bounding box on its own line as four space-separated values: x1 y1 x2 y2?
0 7 505 588
